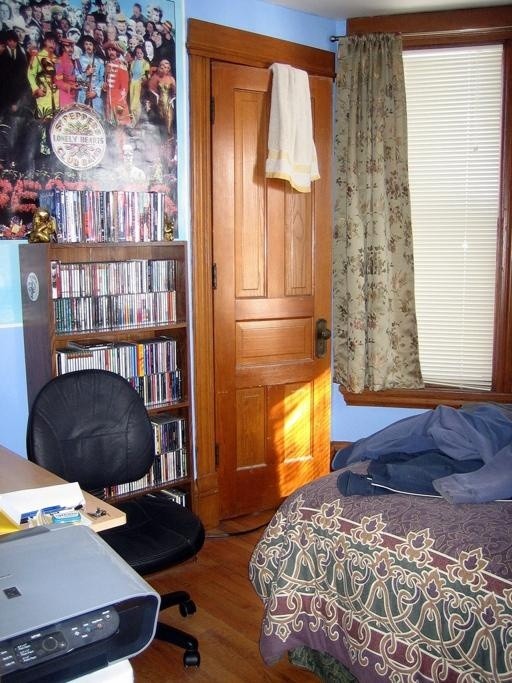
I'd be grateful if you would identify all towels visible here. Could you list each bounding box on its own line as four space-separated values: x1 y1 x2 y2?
264 61 322 196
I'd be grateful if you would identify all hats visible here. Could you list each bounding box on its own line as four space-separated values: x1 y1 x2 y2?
102 40 124 53
114 15 126 22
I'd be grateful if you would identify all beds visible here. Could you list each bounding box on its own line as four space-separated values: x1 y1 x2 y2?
250 456 512 683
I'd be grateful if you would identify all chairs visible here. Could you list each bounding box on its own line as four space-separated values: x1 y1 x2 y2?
25 368 206 670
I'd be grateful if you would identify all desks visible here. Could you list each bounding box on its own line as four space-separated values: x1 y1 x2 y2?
1 445 127 532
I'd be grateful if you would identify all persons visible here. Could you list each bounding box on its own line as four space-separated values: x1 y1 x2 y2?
0 0 177 183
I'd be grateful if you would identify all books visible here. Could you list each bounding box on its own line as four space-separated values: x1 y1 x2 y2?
50 258 189 506
37 187 167 245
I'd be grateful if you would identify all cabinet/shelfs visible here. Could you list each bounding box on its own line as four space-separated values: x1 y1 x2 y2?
18 240 196 566
